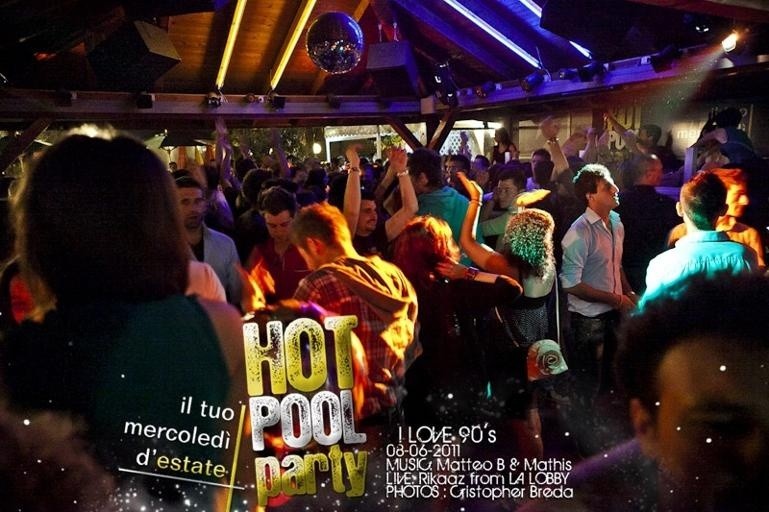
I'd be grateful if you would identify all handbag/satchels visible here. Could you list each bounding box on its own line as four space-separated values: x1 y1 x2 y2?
526 340 568 383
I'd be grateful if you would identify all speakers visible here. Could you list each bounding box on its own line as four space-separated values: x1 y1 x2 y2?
538 0 657 57
86 20 182 91
364 37 424 99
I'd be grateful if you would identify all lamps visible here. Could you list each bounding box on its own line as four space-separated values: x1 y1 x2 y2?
557 68 577 81
206 91 221 106
519 72 544 91
328 93 342 109
651 45 680 73
270 92 286 109
475 82 495 98
57 88 77 100
136 94 152 109
376 95 392 108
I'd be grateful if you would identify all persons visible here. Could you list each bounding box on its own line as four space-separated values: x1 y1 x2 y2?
2 107 768 509
